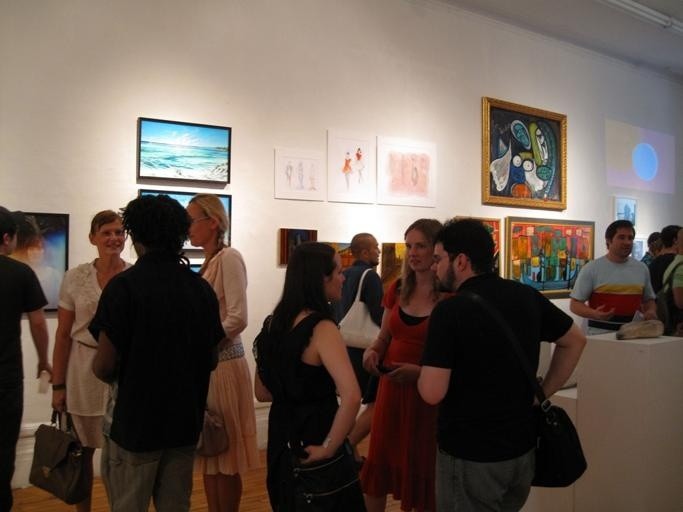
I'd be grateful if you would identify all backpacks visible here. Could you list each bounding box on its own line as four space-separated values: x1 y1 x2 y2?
655 260 683 336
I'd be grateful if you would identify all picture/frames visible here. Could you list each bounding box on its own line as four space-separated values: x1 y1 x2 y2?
186 261 204 273
631 237 647 261
137 188 234 253
454 215 502 268
5 210 72 315
476 94 569 212
135 115 233 188
613 195 637 228
268 127 440 206
501 216 596 300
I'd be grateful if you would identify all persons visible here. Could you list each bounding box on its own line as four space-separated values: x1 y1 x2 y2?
342 147 364 189
283 159 316 191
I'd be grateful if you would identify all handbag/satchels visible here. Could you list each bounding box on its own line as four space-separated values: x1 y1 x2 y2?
336 267 383 350
192 410 230 458
526 405 589 490
27 409 93 506
286 438 367 511
